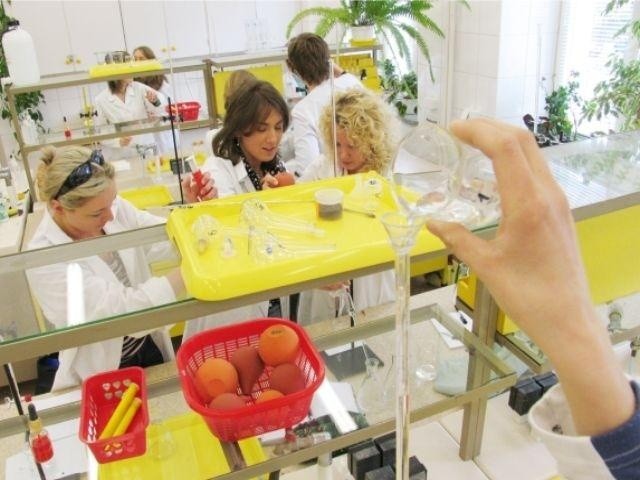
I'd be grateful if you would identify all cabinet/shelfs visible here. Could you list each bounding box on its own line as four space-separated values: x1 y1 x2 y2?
10 1 129 135
416 0 562 153
1 131 640 480
204 0 303 101
7 41 384 202
119 0 211 120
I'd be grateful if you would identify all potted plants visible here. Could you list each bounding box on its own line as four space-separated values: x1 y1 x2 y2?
284 0 472 86
535 70 584 148
386 70 417 117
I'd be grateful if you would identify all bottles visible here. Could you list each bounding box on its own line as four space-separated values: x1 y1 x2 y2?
356 357 388 414
26 403 54 464
1 20 40 86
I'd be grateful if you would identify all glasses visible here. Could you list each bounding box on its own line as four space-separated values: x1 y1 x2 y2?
55 149 104 201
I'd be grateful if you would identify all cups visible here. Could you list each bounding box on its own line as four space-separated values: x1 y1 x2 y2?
415 333 439 379
387 124 501 232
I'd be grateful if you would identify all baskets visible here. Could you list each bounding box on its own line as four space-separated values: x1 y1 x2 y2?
166 102 200 120
177 318 325 443
80 367 148 463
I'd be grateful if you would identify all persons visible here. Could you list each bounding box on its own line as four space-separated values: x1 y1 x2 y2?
92 49 167 162
293 86 406 329
274 31 361 181
425 116 640 480
132 46 181 156
181 79 352 344
23 145 185 393
222 71 261 112
